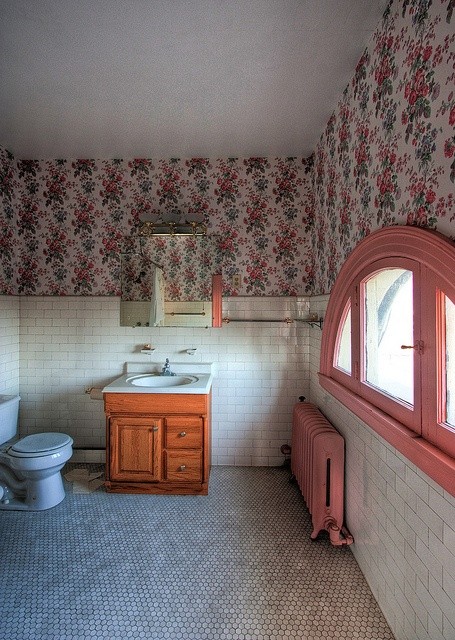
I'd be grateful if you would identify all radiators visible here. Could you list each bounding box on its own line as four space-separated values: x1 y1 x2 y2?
289 395 354 547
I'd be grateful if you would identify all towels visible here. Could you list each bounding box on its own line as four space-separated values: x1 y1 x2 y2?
149 267 166 329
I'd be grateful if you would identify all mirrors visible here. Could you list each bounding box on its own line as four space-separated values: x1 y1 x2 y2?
119 237 223 328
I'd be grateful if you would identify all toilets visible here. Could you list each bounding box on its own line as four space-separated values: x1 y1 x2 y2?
0 394 74 511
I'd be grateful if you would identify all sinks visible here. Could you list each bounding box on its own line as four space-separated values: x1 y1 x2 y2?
127 375 198 388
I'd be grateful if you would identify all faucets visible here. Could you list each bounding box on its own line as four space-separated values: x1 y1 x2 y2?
161 359 178 377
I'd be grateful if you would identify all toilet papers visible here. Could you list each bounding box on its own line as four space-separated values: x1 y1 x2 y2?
88 387 104 399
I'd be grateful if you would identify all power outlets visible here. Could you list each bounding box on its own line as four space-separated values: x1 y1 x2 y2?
232 275 240 289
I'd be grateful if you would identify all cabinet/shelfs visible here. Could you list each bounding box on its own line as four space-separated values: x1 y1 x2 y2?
104 395 213 494
